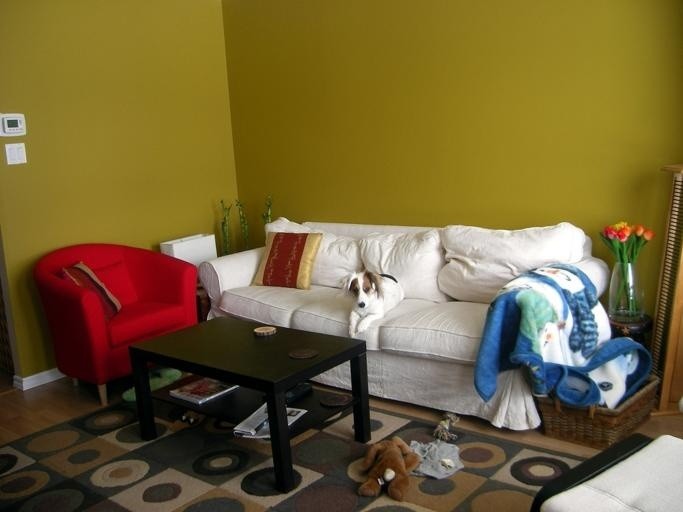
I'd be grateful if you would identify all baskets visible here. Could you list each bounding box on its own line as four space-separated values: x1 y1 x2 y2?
530 374 661 447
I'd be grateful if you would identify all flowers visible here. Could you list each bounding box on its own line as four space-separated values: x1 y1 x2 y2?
601 220 655 315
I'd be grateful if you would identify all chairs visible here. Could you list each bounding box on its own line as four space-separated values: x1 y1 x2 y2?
31 242 199 410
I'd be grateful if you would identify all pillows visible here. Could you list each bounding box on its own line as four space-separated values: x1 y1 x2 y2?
436 222 585 303
60 259 122 319
263 217 363 289
249 231 322 289
358 229 451 303
93 262 138 306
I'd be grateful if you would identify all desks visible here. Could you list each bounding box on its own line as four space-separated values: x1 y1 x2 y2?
608 315 653 354
195 282 210 323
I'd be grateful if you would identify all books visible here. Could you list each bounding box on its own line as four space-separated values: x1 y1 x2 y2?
167 377 240 406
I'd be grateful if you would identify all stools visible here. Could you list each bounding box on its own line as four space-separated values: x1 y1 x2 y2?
532 433 683 512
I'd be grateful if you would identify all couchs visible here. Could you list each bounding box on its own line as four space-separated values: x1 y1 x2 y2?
196 222 610 432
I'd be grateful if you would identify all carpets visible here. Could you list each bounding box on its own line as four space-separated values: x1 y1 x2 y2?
0 376 587 512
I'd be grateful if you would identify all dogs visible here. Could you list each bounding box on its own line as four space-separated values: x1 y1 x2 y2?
341 268 404 340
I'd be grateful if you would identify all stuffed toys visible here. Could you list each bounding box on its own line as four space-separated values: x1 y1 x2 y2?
355 435 421 502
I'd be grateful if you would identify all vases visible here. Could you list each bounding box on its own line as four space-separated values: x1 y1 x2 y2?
607 261 647 323
